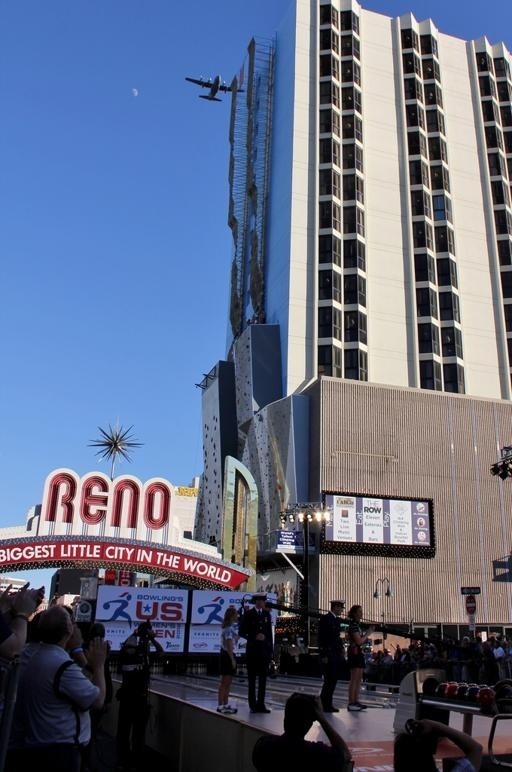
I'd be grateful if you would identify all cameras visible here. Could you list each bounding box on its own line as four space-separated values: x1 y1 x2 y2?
404 718 425 735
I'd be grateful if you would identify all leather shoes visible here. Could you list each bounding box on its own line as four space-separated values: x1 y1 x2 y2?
250 707 256 713
256 707 270 713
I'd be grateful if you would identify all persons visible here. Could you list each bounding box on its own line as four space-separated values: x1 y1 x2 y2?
116 627 166 771
251 692 354 772
316 598 347 713
346 604 377 712
277 632 511 686
216 606 246 710
236 593 277 715
0 582 113 771
392 718 484 772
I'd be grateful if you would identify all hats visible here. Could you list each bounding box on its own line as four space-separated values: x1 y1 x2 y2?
251 595 267 604
329 600 345 608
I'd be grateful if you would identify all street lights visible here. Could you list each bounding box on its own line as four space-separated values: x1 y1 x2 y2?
280 502 330 646
372 577 394 651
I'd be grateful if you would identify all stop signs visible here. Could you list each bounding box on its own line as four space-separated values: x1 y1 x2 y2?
466 596 476 615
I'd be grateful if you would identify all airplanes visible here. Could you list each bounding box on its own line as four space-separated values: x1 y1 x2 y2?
185 74 231 102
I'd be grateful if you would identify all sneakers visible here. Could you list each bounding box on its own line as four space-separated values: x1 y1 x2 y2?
348 702 367 711
323 708 339 712
225 708 237 714
217 707 228 713
